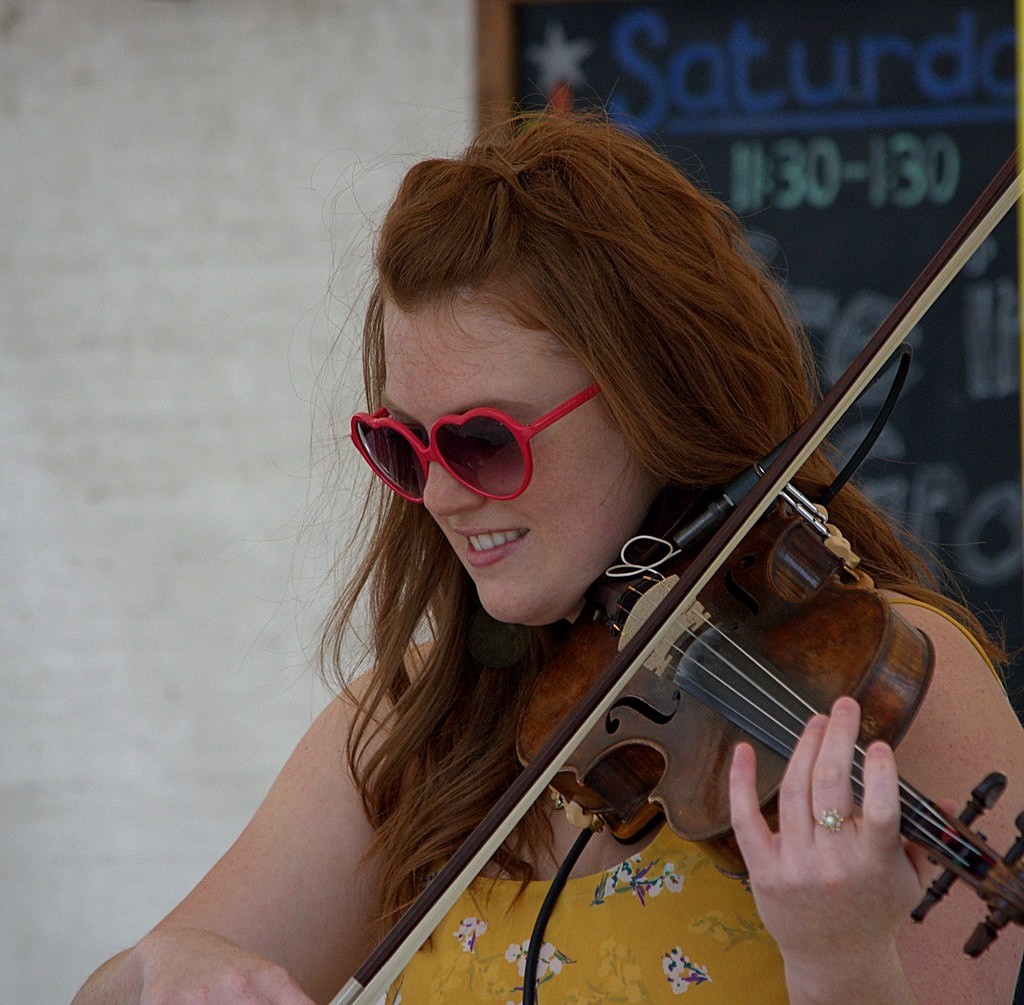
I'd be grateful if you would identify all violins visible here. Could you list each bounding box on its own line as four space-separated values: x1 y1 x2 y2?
513 471 1023 958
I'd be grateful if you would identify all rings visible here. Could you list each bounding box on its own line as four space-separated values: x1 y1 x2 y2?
813 809 853 833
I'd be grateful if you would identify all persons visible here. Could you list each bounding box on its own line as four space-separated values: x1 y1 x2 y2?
68 107 1024 1005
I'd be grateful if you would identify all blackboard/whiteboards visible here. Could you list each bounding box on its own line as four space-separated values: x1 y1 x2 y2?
471 0 1024 724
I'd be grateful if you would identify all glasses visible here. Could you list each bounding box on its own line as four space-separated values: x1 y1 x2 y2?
351 380 602 503
